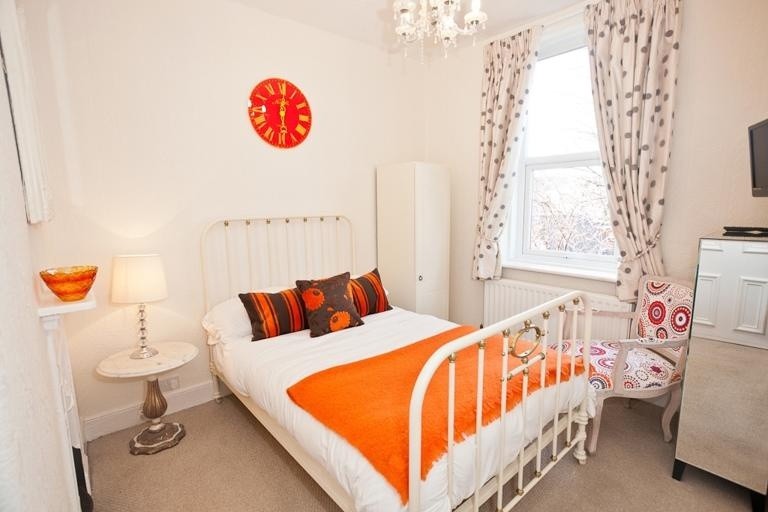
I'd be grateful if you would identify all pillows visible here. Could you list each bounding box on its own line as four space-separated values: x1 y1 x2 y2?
291 272 366 339
236 289 307 342
343 268 393 320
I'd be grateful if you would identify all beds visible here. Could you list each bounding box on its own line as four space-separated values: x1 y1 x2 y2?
198 217 593 511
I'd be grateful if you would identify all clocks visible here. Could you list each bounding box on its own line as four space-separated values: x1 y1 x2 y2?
241 72 319 155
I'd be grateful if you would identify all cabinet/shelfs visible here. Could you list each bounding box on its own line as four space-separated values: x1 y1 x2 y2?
690 240 767 350
373 162 450 317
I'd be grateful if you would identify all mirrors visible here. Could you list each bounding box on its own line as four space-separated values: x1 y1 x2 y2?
675 238 767 496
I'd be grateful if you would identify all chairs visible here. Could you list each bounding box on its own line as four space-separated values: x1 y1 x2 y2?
549 275 696 456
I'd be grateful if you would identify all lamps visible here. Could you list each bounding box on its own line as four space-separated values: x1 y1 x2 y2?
109 253 170 359
388 1 489 64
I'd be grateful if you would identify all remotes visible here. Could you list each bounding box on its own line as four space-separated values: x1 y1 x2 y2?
723 225 768 232
722 232 768 237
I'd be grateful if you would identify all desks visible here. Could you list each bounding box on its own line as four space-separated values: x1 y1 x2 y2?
96 343 201 456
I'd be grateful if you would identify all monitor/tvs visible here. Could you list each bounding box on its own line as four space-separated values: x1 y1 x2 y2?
747 119 768 197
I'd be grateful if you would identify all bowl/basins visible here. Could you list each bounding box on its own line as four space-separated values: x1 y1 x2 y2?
37 265 98 303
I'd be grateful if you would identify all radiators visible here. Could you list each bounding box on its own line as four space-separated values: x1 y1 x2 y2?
483 281 631 349
